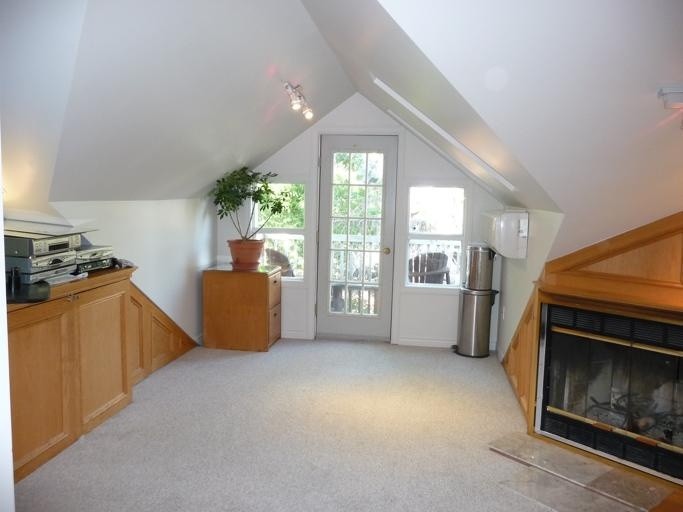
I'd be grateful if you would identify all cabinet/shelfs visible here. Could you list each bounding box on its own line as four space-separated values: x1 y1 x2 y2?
202 266 283 354
7 264 140 485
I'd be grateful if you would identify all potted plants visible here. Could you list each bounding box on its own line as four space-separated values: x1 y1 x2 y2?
207 167 292 271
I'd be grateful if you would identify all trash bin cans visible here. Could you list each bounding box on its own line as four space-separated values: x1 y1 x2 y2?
466 245 496 290
456 290 492 358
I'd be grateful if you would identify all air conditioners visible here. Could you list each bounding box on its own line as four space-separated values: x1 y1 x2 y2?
478 206 530 260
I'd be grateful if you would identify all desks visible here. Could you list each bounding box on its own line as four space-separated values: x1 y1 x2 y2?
330 283 378 313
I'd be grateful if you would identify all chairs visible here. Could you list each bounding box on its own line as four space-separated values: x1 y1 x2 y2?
408 253 451 284
266 249 296 279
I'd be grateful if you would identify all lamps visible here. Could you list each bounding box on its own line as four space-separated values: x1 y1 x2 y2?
282 81 315 121
